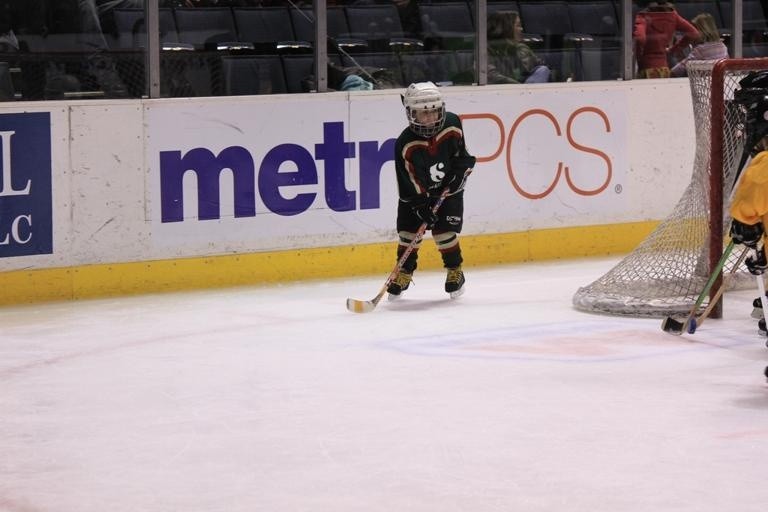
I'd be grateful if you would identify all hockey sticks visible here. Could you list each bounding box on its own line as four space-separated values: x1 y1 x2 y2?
661 237 749 336
346 187 449 313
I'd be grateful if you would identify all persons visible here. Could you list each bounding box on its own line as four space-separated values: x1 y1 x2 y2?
117 17 192 96
734 69 768 334
631 1 700 78
666 12 743 77
475 10 556 84
0 0 20 66
728 93 768 382
22 58 83 103
386 81 476 299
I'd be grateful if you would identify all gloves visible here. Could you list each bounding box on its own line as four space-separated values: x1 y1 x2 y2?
408 196 435 225
441 169 464 193
745 250 768 275
729 219 764 247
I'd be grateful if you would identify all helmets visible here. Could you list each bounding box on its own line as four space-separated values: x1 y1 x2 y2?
403 80 446 138
732 70 768 158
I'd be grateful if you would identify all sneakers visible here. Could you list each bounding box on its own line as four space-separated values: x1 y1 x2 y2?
445 268 465 292
387 271 412 295
